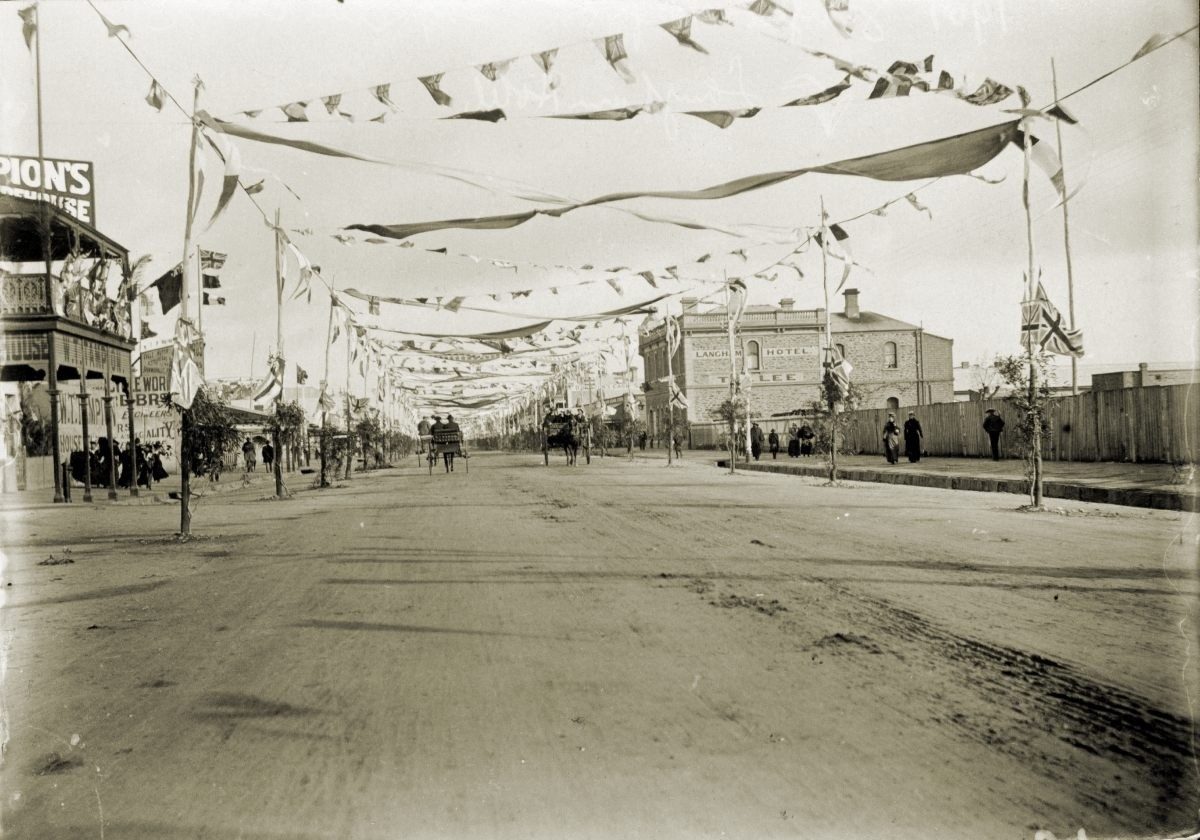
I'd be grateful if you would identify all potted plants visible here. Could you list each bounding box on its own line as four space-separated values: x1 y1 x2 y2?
171 391 239 476
993 354 1058 448
318 417 416 472
544 378 867 471
268 401 302 446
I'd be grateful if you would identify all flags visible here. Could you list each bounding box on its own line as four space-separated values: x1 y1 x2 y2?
18 1 1166 413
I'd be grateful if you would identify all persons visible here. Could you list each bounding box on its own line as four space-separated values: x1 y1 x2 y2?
672 427 684 457
883 412 901 465
69 434 170 488
241 437 256 472
262 439 275 473
904 410 924 462
797 420 815 457
768 428 780 461
418 414 462 445
787 422 801 458
982 408 1004 461
751 421 764 461
543 407 587 425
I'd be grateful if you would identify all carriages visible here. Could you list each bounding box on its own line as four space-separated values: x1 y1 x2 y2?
417 435 441 468
426 429 470 474
540 417 591 467
